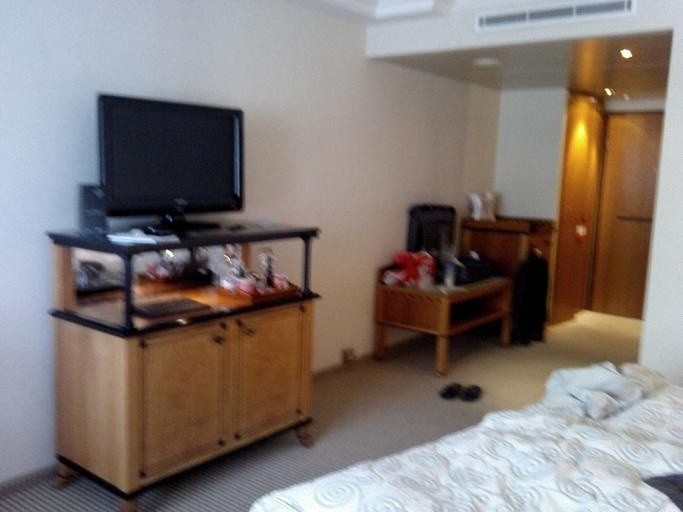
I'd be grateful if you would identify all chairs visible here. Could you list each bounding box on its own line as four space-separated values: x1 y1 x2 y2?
404 204 458 257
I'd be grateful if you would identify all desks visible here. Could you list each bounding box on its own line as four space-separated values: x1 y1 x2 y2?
460 212 554 278
373 271 513 377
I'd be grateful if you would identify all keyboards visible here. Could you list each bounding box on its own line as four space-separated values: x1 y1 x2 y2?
131 298 211 319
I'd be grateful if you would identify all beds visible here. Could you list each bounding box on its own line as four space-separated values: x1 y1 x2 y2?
252 362 681 512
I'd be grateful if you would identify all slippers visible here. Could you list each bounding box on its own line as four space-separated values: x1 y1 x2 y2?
437 381 481 402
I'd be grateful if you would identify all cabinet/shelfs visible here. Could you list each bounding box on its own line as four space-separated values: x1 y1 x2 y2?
45 218 322 512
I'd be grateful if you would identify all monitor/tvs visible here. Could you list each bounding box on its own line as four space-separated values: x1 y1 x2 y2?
99 94 242 236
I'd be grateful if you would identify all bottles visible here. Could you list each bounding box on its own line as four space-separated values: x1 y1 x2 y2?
257 244 277 292
443 260 455 289
220 244 241 299
